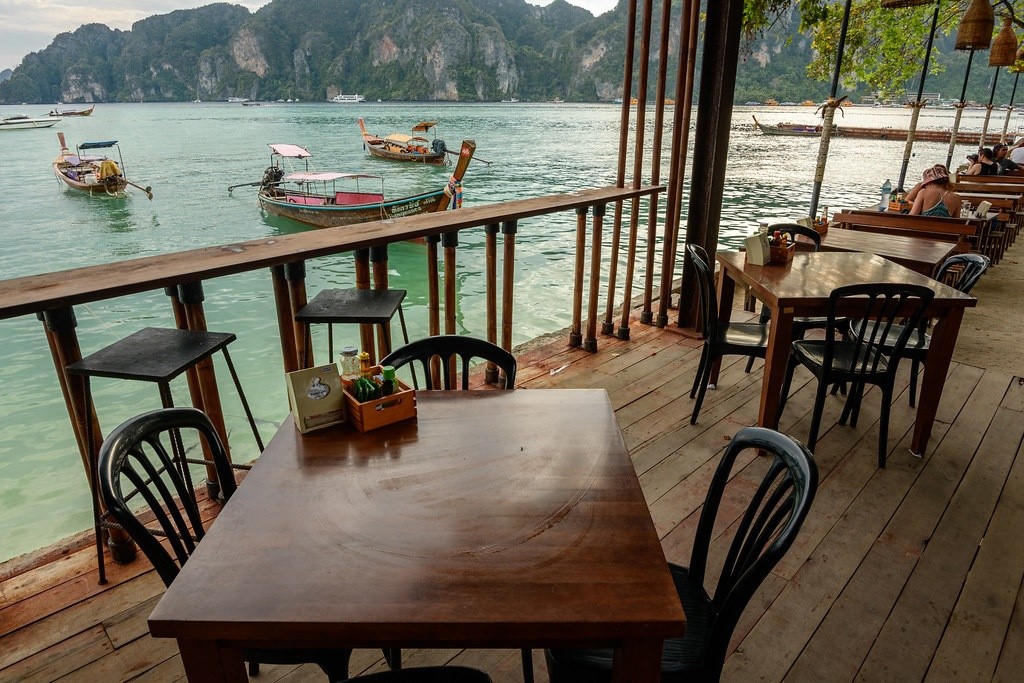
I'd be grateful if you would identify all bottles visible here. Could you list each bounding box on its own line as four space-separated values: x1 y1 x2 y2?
340 345 360 389
759 222 788 249
359 351 373 381
879 178 891 212
891 195 908 203
382 366 399 393
814 206 828 233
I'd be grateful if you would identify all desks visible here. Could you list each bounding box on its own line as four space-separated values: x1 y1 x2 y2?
701 251 977 458
148 388 689 683
293 288 419 390
767 176 1024 280
63 326 265 584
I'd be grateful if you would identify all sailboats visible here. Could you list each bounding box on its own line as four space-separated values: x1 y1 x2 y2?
58 96 63 105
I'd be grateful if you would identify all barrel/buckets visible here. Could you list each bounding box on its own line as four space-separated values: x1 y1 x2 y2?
85 174 97 184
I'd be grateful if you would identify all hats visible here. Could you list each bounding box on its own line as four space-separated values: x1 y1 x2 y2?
920 166 948 188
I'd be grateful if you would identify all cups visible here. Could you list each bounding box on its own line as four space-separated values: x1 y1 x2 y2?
961 202 971 217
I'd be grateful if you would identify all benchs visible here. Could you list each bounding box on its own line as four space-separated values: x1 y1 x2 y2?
926 204 1024 328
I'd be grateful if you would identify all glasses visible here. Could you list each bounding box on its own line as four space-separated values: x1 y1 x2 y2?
998 142 1008 148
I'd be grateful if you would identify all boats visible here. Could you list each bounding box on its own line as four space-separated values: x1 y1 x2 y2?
745 98 853 107
228 97 260 106
872 95 1024 115
358 117 448 166
49 104 95 116
612 94 638 105
191 92 201 103
0 113 61 129
276 89 302 103
500 98 519 103
753 115 838 136
548 97 564 103
50 132 129 192
325 88 366 102
258 140 476 245
664 99 676 105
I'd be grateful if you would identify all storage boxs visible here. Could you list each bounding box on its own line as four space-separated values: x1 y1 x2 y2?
341 365 419 432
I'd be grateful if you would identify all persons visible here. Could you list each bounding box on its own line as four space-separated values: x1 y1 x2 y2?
905 164 954 203
965 148 1002 175
993 143 1024 176
1010 142 1024 163
908 167 960 218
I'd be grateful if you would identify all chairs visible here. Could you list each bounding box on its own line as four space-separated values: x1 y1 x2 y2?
744 223 847 379
771 282 934 470
369 334 519 667
687 243 770 425
840 254 990 429
98 407 406 683
544 426 818 683
